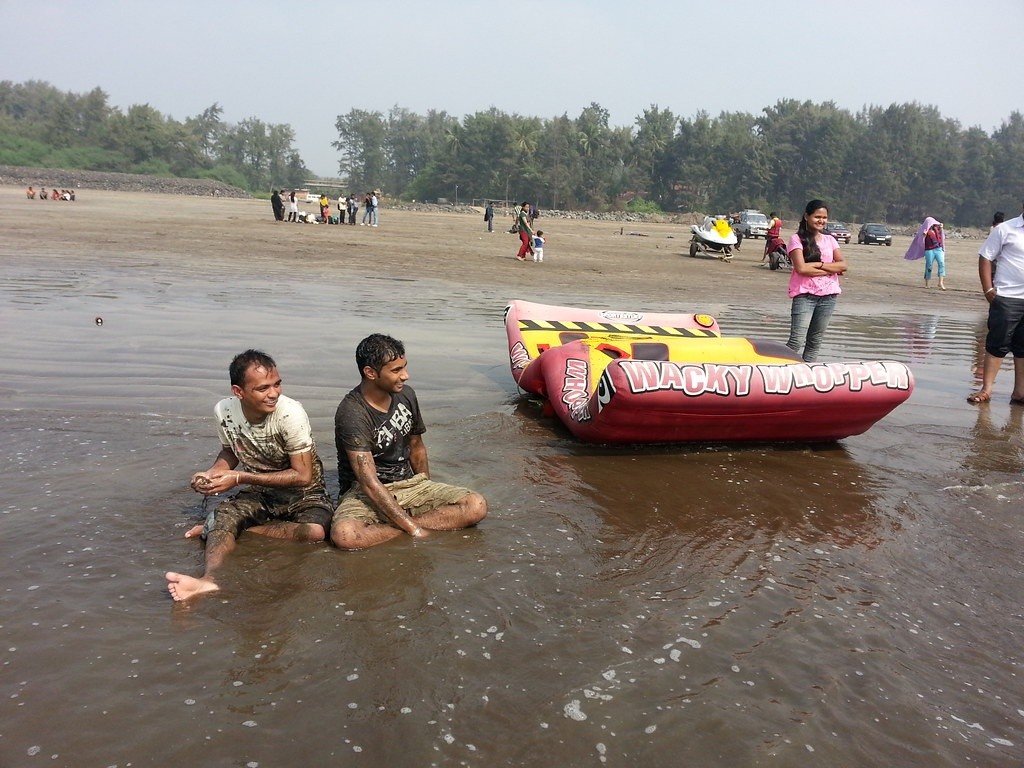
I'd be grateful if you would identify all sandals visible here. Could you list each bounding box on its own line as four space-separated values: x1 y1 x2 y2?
967 390 992 402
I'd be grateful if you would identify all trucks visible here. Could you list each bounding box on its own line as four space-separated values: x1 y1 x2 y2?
728 210 769 238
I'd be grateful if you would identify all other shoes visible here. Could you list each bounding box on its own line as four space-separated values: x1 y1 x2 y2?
925 286 930 288
275 220 298 224
517 249 527 261
489 230 495 232
339 222 378 227
938 282 946 290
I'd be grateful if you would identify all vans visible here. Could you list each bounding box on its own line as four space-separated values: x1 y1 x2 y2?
305 194 322 201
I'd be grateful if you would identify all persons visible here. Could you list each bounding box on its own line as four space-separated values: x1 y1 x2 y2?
786 199 849 363
26 186 75 201
966 213 1024 403
729 211 781 260
922 212 1004 290
327 333 489 551
486 202 546 263
271 190 378 228
165 350 333 603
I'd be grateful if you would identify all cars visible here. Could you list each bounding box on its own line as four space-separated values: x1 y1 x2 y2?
856 221 891 245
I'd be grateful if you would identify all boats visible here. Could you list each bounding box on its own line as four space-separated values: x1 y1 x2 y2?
504 299 915 446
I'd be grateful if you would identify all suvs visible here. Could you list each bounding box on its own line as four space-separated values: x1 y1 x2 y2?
821 223 852 244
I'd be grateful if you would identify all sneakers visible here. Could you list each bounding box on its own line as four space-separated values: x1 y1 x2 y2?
533 259 543 263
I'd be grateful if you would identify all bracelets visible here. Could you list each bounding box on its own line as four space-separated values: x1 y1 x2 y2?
985 288 994 294
819 262 824 269
411 527 421 537
235 471 239 486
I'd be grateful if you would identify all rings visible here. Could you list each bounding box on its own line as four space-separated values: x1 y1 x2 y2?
215 493 218 496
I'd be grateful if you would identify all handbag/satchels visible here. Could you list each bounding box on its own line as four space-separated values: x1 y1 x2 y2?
298 212 338 224
368 207 374 212
484 214 489 222
